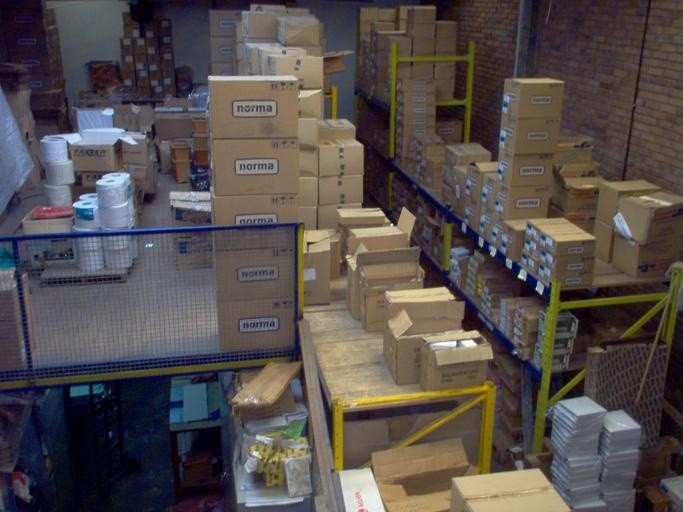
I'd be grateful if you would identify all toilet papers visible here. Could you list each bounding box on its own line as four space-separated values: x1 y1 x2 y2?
40 131 139 271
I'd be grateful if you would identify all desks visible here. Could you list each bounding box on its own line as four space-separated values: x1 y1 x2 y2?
170 377 227 499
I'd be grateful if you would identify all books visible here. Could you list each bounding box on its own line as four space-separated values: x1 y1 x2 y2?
659 474 683 512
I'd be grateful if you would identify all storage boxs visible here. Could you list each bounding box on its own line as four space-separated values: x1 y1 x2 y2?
465 75 682 512
0 1 213 273
204 1 464 512
